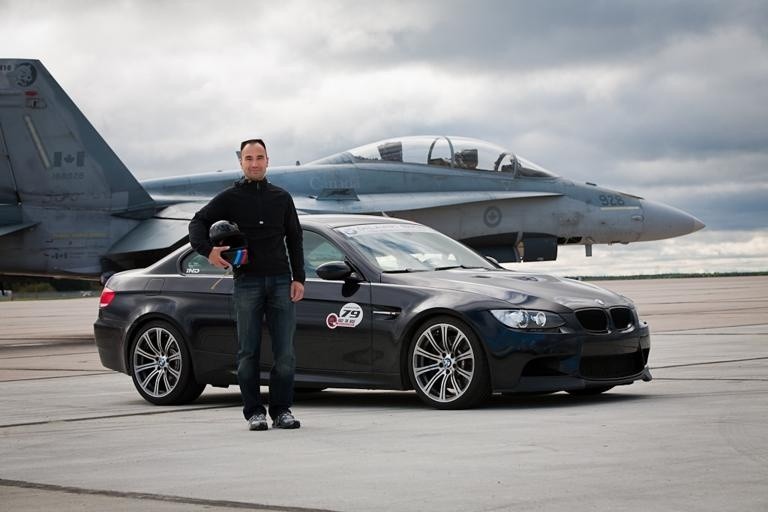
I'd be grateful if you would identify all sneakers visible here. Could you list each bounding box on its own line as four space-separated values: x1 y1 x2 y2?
271 412 300 429
248 414 268 430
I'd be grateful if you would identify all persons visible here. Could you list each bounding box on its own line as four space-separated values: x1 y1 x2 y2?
188 139 305 431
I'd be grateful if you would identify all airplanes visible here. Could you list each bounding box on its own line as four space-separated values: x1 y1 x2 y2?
0 57 707 294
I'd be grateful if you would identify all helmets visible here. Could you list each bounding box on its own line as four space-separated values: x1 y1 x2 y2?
209 219 248 267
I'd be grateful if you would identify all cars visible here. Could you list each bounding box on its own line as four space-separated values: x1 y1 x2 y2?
93 211 653 409
